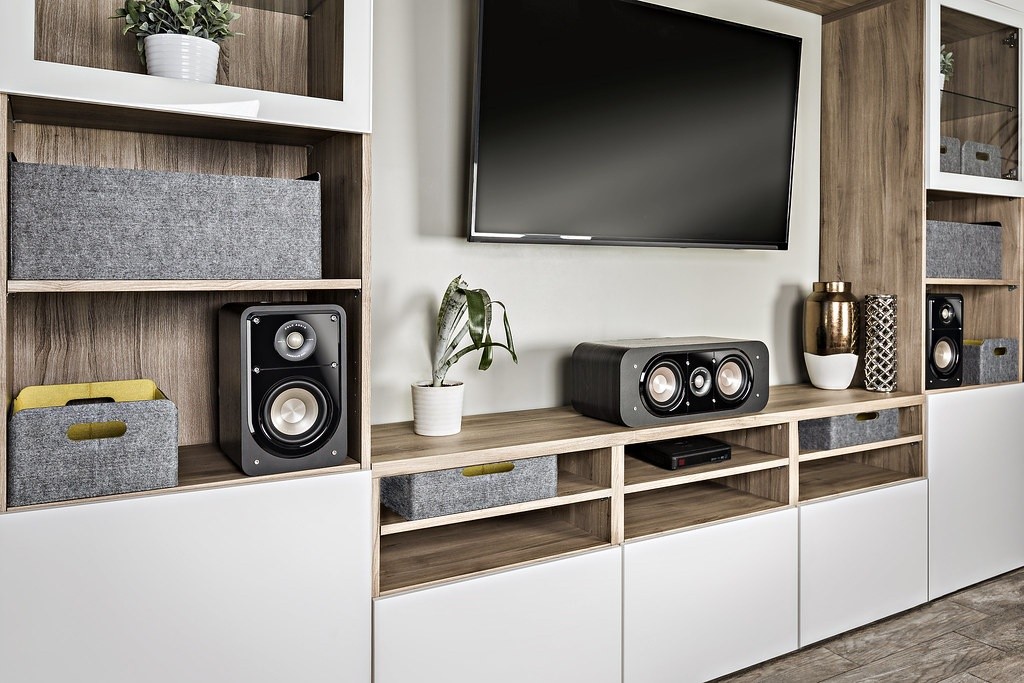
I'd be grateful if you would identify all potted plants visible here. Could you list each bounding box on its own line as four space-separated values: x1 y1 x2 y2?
940 43 954 90
411 275 519 436
108 0 247 84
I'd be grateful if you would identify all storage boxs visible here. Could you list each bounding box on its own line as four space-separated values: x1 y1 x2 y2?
940 135 962 174
926 220 1002 278
797 408 901 452
963 338 1018 384
8 151 322 281
961 140 1002 179
379 454 559 520
8 378 179 507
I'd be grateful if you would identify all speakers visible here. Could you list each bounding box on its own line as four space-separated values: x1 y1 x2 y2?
572 336 769 428
924 292 963 390
220 301 349 477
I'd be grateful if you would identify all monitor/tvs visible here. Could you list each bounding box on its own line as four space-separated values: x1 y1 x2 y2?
466 0 802 250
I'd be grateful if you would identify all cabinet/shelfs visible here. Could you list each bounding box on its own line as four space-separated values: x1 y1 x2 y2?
373 383 927 683
0 0 374 683
818 0 1024 604
